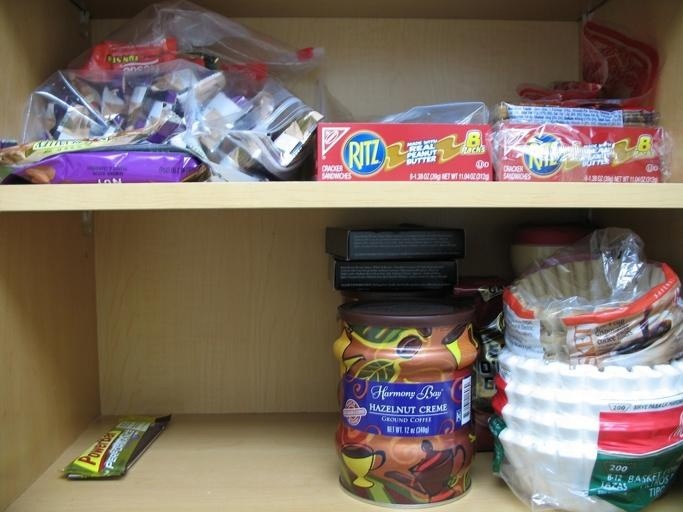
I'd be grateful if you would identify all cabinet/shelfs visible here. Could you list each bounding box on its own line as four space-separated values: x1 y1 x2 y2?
0 0 683 510
